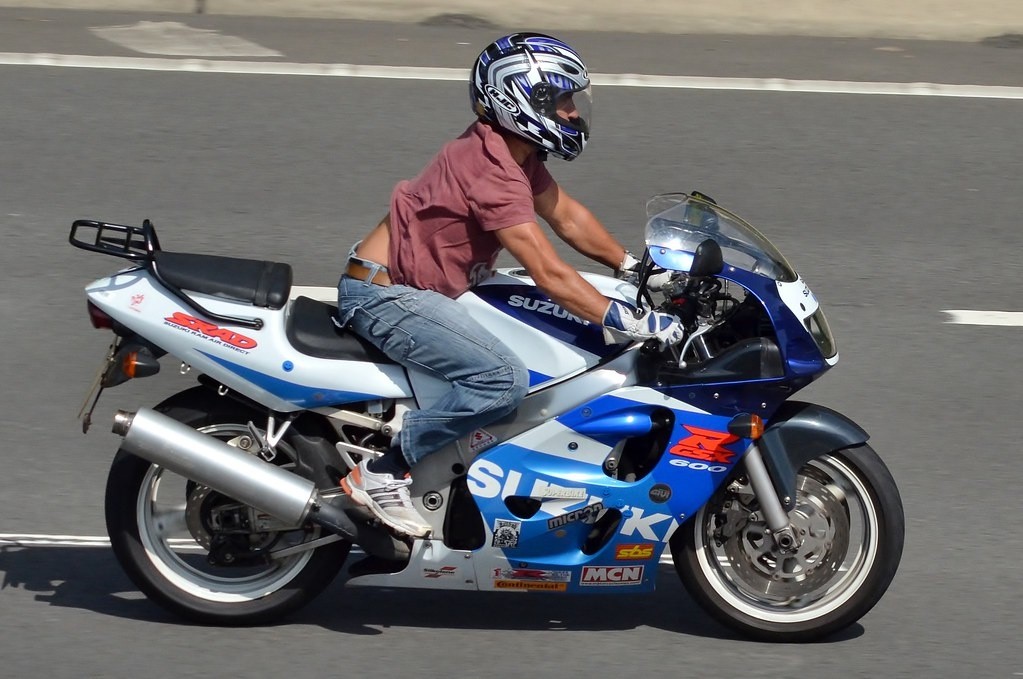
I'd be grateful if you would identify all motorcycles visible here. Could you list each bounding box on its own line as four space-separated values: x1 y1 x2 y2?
68 186 909 646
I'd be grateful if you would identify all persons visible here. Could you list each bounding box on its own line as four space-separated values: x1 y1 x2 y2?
331 32 684 539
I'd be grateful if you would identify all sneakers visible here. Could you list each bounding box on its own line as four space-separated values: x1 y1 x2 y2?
340 458 432 539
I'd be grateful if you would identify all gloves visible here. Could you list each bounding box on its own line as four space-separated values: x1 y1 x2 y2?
602 300 685 352
614 250 690 292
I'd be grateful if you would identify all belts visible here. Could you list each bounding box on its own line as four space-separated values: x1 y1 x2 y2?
345 263 392 287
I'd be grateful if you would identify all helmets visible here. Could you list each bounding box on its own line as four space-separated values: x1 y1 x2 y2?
469 32 594 161
